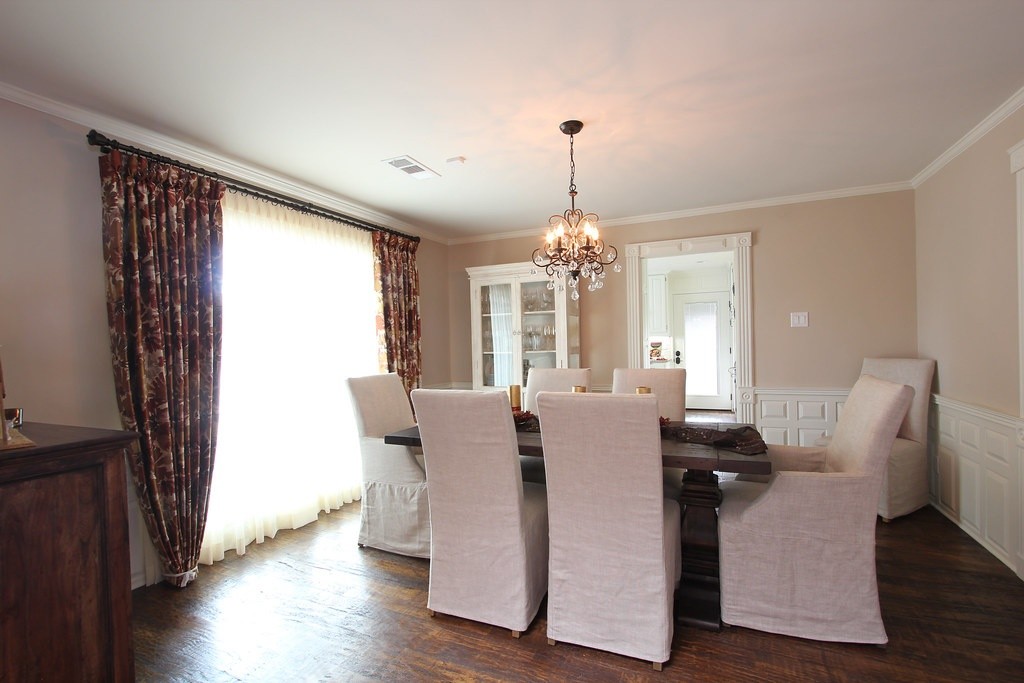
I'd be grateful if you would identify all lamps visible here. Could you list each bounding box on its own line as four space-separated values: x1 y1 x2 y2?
533 120 624 303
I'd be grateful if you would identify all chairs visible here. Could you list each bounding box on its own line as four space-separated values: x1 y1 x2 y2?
816 356 936 523
536 390 683 671
612 367 687 513
717 373 917 649
344 370 431 558
518 368 591 482
410 388 549 639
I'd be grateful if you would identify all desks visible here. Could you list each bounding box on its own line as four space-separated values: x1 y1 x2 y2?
384 422 773 631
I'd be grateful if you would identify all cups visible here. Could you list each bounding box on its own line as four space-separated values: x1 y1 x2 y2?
527 325 555 350
522 288 554 311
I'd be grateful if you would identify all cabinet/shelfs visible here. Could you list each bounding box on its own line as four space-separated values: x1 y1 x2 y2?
463 259 581 410
649 275 672 337
1 421 142 683
